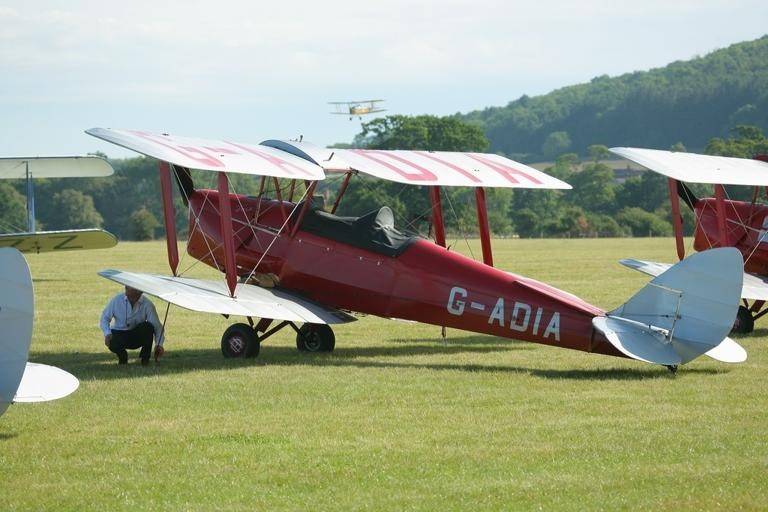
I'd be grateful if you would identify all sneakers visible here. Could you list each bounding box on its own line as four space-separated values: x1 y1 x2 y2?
118 358 128 364
141 357 150 365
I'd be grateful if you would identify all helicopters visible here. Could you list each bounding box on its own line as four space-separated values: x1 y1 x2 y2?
328 98 387 121
607 145 768 333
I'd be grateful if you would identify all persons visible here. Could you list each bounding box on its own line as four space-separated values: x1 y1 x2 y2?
98 285 166 366
